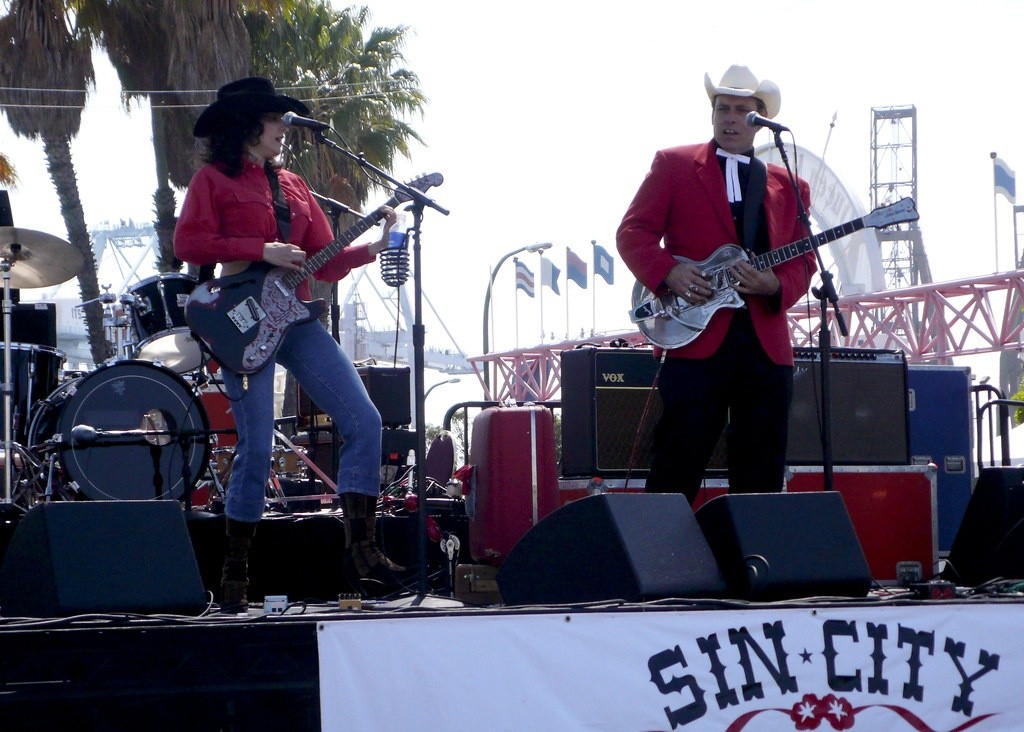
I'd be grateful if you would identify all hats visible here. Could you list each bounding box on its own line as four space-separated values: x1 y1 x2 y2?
704 64 781 131
194 77 310 138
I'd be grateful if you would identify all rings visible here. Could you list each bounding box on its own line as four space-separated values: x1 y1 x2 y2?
685 290 691 297
702 271 706 277
689 283 698 290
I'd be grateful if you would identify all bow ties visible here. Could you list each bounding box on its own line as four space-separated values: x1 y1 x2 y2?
716 148 751 204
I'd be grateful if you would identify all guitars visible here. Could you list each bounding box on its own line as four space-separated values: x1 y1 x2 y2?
628 195 918 351
181 170 444 377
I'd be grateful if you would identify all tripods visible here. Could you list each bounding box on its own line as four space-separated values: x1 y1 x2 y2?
0 255 75 518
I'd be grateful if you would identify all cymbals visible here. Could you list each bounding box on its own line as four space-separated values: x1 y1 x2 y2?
0 224 85 290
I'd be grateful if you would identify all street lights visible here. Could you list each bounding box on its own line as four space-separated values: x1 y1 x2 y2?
481 241 552 407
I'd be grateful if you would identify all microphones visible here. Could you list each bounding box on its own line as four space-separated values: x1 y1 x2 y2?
745 110 791 131
70 424 104 443
282 111 331 130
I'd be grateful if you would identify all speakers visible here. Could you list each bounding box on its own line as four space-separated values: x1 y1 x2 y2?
289 366 416 493
495 491 871 608
0 501 210 619
558 347 733 478
787 345 911 468
943 465 1024 591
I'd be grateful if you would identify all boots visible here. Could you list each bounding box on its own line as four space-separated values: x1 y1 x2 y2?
336 493 406 578
219 518 258 614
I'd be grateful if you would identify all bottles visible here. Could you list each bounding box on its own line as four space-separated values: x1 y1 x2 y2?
384 214 410 284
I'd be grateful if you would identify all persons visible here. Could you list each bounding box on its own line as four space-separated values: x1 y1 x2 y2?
613 64 812 502
171 76 407 613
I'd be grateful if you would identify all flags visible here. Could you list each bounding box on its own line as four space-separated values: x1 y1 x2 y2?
566 249 587 289
594 244 614 285
993 158 1016 204
516 261 535 298
541 258 561 295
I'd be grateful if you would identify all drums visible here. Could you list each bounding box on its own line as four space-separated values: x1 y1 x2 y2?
28 356 213 504
125 271 212 376
0 340 69 466
